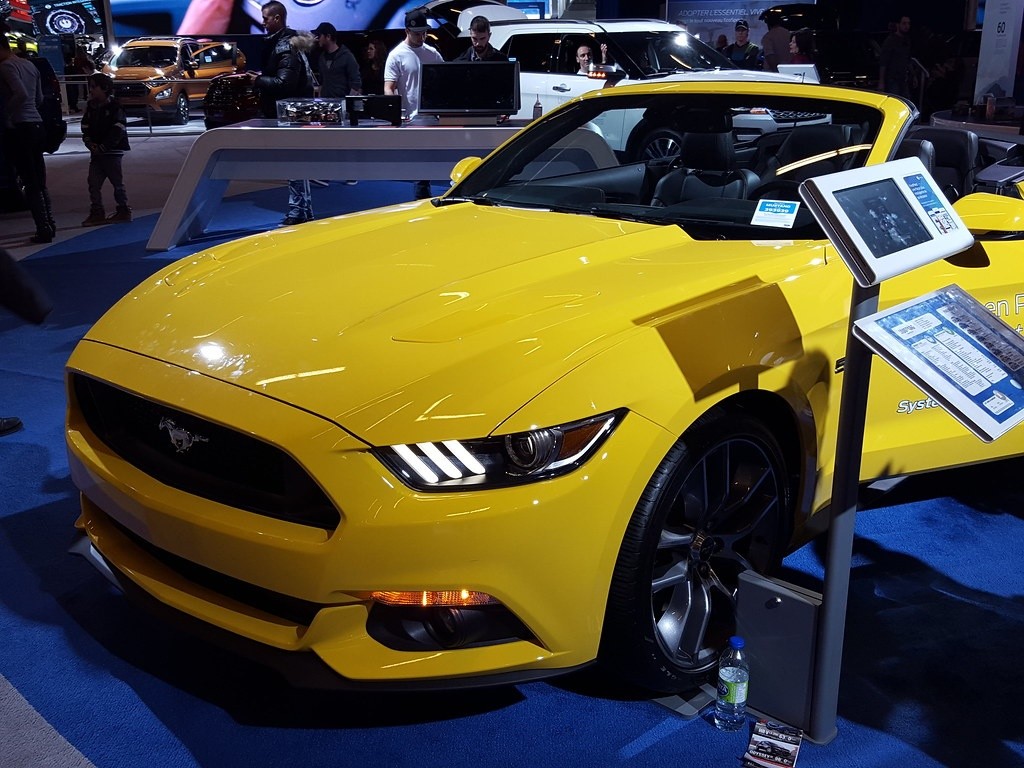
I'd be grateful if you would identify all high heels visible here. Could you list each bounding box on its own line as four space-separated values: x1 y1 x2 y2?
30 222 57 242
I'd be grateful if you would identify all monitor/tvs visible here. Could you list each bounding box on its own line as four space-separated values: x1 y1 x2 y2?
417 60 521 116
832 178 934 258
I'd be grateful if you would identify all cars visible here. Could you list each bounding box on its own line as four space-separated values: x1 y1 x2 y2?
5 31 37 57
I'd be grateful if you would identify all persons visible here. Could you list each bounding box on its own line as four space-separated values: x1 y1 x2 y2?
359 42 385 95
80 73 131 226
789 31 823 82
760 13 791 73
0 33 56 243
15 40 103 103
576 44 607 76
880 17 917 100
452 16 509 63
715 34 728 54
722 20 759 71
384 12 445 123
245 1 314 119
310 22 361 98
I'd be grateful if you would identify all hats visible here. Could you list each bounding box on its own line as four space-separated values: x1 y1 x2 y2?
311 22 336 37
405 12 431 32
735 19 748 30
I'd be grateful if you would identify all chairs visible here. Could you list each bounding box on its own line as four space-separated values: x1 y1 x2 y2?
651 124 984 212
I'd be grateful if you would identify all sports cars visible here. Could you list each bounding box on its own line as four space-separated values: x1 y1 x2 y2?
63 82 1024 694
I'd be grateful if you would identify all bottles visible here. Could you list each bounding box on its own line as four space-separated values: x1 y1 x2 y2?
714 636 750 732
533 94 542 121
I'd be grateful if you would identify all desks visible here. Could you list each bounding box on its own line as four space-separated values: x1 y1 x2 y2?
145 118 618 250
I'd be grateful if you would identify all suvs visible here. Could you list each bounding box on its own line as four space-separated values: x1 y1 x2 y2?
449 16 832 166
99 35 247 126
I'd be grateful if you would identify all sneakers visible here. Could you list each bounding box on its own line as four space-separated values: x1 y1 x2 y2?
106 208 132 224
81 214 107 227
